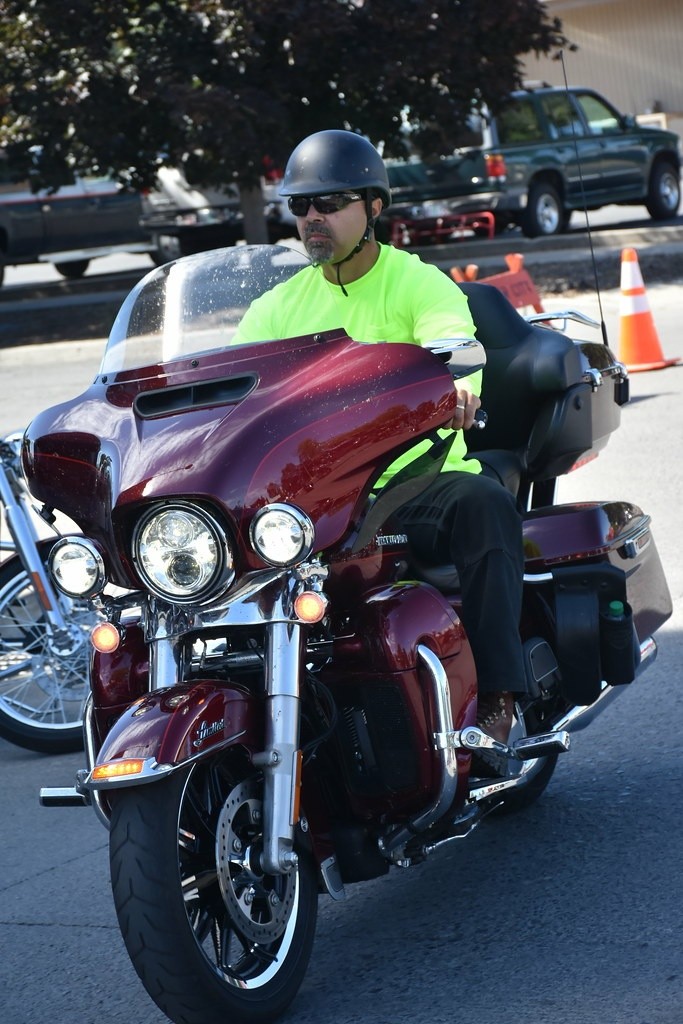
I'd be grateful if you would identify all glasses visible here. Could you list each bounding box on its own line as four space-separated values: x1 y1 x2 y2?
288 191 364 215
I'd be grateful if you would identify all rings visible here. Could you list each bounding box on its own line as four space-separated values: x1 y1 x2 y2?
456 404 466 410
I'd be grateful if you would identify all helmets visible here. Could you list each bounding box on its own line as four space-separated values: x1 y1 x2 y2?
278 129 392 209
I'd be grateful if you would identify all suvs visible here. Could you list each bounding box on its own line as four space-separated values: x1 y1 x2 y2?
136 152 308 252
375 81 683 238
0 136 199 287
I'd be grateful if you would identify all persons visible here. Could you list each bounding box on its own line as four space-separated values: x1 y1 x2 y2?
227 127 528 778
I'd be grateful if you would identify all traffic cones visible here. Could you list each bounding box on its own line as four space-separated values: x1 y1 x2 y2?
610 247 682 372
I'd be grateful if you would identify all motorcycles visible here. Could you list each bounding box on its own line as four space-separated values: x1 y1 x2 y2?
0 430 129 755
20 244 677 1024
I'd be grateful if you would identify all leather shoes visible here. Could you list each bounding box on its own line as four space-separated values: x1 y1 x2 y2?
472 688 514 779
